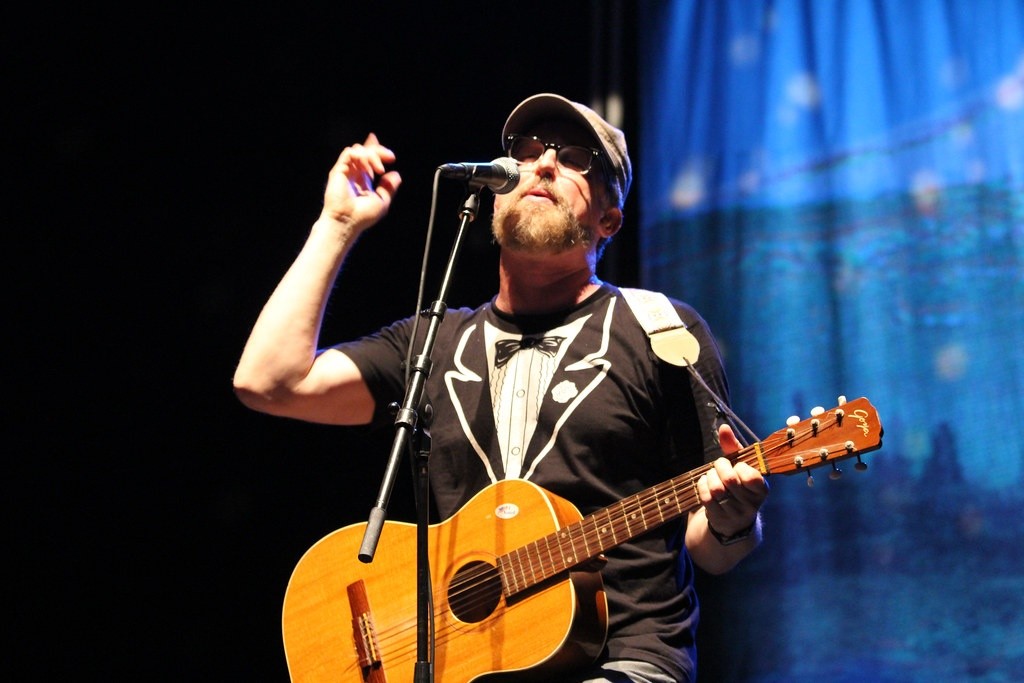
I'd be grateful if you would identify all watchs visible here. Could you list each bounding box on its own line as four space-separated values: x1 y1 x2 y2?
707 521 758 546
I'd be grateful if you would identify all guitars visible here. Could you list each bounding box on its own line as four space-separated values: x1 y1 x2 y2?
280 394 884 683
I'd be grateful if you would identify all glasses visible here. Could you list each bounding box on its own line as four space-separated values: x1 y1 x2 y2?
507 135 615 207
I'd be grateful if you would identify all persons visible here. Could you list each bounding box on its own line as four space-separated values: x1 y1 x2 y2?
234 90 770 683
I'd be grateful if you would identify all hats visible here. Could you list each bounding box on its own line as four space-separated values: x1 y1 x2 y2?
501 92 633 201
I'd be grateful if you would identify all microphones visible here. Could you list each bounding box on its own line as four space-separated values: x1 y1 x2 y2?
438 157 520 194
